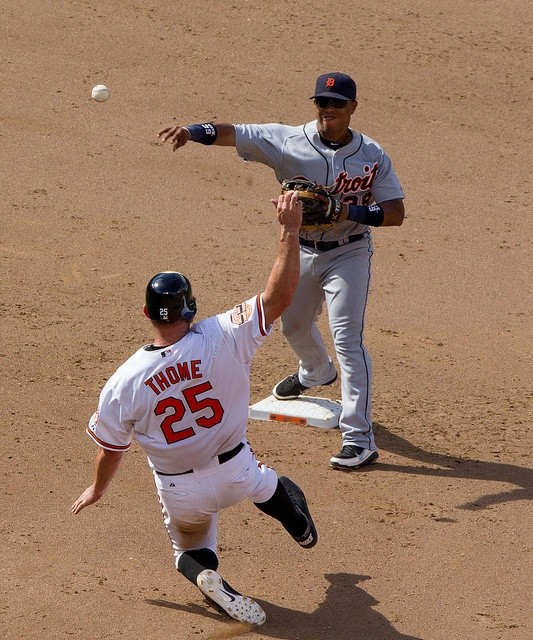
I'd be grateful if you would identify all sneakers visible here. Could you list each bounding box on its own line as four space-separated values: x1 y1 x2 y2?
330 441 379 471
279 477 317 550
195 569 266 625
273 369 336 400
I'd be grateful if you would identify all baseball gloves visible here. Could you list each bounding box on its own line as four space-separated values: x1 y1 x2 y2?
281 179 343 233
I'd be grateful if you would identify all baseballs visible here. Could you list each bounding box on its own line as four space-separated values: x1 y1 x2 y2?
91 84 109 102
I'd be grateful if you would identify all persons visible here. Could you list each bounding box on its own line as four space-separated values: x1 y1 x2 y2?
154 72 407 472
68 189 319 628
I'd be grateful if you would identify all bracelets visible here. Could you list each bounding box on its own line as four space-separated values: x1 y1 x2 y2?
182 123 217 145
346 204 384 227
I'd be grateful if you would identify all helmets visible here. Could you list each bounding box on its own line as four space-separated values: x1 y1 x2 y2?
146 271 195 324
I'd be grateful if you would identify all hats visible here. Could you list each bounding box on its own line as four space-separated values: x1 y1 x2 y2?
308 73 357 103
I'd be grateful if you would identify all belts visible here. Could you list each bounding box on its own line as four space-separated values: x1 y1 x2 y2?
298 235 365 251
154 442 244 477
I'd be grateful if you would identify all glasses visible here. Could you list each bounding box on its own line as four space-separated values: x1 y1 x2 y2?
313 98 350 110
